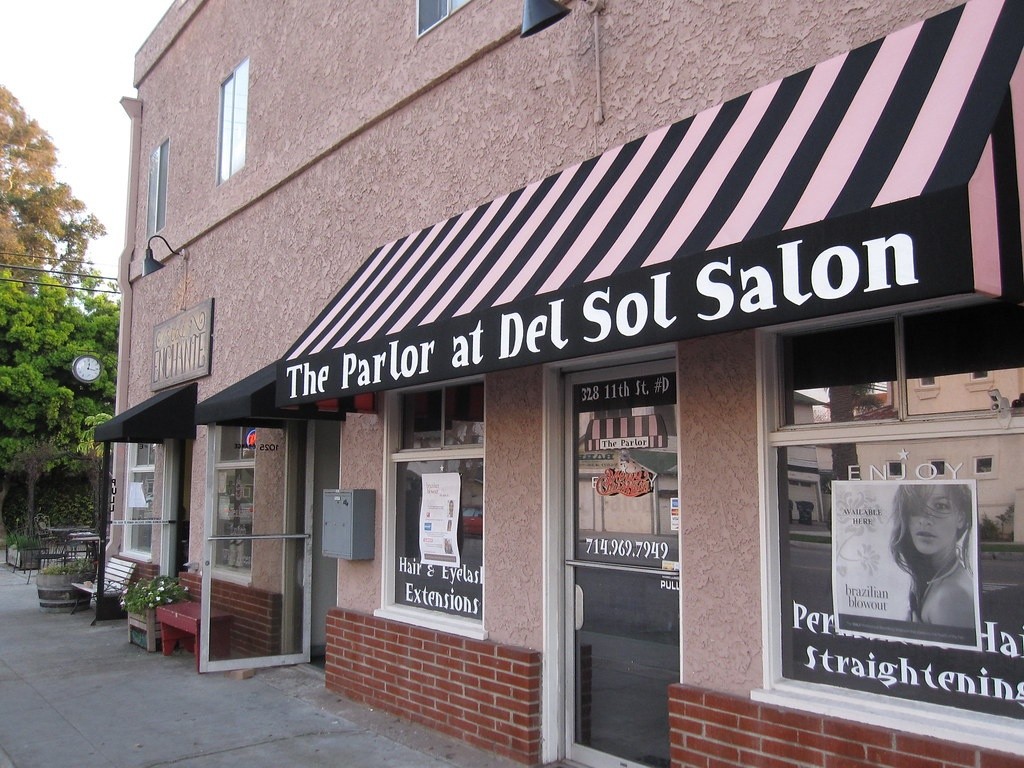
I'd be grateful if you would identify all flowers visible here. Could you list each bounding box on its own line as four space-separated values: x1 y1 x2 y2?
117 574 189 611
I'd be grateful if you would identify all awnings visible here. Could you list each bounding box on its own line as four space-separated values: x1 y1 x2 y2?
94 382 198 444
195 362 284 428
276 0 1024 414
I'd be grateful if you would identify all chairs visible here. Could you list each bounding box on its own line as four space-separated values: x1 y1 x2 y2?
27 533 70 585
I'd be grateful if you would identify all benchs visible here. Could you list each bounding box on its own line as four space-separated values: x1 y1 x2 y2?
70 557 137 625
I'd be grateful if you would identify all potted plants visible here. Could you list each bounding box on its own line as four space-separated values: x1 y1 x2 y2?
35 559 95 613
6 535 41 569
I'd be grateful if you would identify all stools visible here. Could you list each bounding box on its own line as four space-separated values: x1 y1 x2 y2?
156 603 233 674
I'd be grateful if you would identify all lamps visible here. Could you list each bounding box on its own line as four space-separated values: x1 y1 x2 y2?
518 0 603 39
141 234 189 277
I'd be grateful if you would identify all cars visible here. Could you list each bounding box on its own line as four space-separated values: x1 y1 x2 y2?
461 504 483 538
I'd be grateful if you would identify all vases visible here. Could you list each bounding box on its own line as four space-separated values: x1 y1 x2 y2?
125 607 162 651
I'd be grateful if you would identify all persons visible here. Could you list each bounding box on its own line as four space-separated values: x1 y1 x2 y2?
890 484 975 629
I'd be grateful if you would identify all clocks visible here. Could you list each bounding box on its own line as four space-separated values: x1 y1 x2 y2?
72 354 103 383
